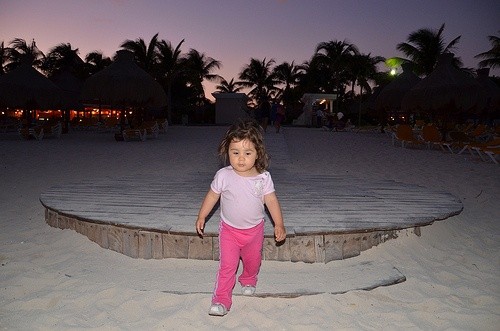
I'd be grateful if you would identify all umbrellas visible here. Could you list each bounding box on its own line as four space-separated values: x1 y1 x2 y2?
377 63 499 109
0 60 169 110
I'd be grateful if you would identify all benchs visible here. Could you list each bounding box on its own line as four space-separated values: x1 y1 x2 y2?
122 119 169 143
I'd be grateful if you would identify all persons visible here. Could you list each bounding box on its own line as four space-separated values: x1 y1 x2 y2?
261 98 271 131
272 98 284 133
118 118 133 131
46 113 81 130
337 111 343 120
196 119 286 315
317 107 324 128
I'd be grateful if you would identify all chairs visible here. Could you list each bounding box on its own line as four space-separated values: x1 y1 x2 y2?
384 120 500 165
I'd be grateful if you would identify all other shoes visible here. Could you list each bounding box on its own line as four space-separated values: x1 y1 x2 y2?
208 303 228 316
242 285 256 296
